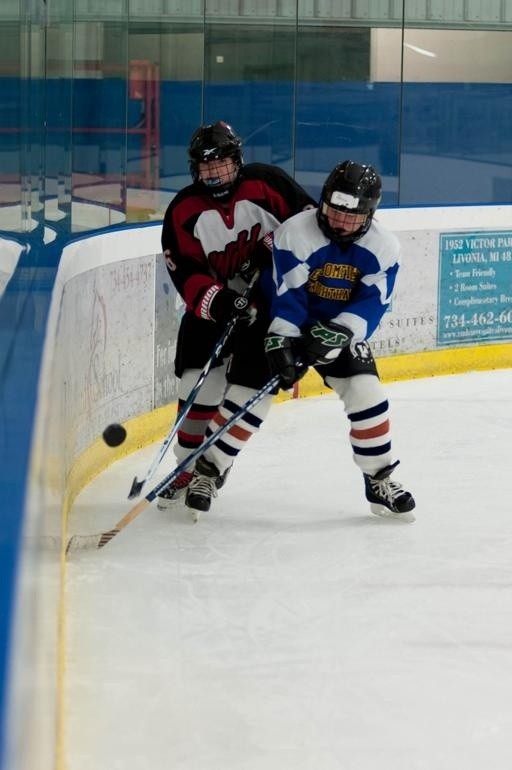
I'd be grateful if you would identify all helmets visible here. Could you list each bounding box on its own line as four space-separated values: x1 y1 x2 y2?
318 159 382 235
186 121 243 199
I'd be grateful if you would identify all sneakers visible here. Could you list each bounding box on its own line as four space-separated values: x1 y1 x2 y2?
158 469 193 499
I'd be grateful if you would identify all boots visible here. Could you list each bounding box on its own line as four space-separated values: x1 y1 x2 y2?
364 460 415 512
185 456 219 512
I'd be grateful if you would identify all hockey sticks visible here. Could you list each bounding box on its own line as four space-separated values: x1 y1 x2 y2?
65 359 302 558
127 259 262 500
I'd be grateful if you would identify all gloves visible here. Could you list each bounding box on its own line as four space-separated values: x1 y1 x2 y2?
263 337 305 391
241 237 272 287
308 320 354 367
210 287 252 320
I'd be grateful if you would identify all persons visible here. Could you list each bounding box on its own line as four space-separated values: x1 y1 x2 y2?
153 120 321 501
185 157 416 513
